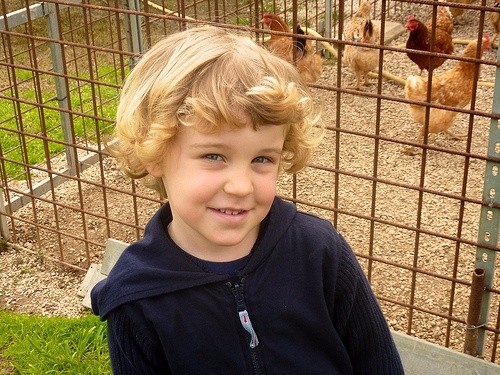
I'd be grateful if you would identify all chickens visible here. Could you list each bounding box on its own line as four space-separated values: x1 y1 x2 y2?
488 0 500 50
344 1 381 91
257 11 322 84
405 33 491 156
404 0 455 76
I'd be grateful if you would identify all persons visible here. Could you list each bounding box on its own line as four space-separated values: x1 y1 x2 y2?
87 26 407 375
